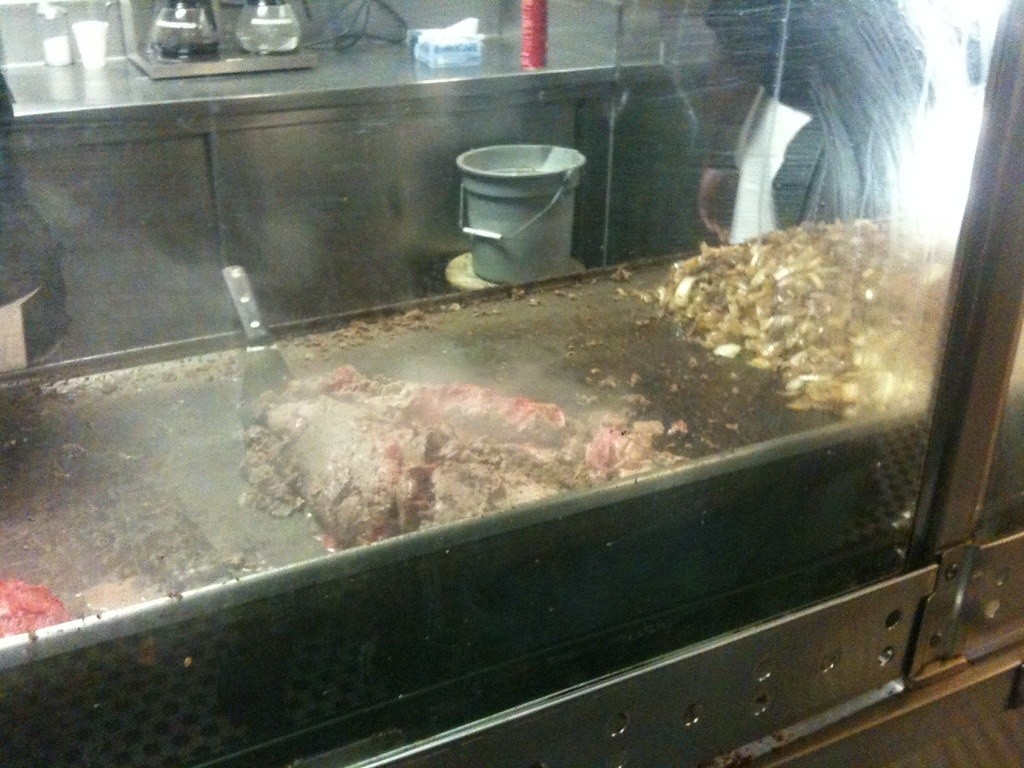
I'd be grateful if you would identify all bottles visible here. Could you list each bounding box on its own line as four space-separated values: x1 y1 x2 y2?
520 0 548 70
152 0 214 59
235 1 301 54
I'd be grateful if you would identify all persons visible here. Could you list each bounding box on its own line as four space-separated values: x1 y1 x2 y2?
696 0 929 246
0 74 67 373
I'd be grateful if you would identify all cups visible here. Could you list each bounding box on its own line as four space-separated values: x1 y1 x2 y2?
73 20 108 67
37 15 72 67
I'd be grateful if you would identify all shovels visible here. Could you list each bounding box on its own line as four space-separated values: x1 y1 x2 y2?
222 264 301 449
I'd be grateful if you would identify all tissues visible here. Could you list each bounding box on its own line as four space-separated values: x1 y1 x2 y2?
413 16 487 69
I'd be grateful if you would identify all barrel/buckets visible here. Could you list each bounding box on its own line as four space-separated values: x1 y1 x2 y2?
456 144 587 284
